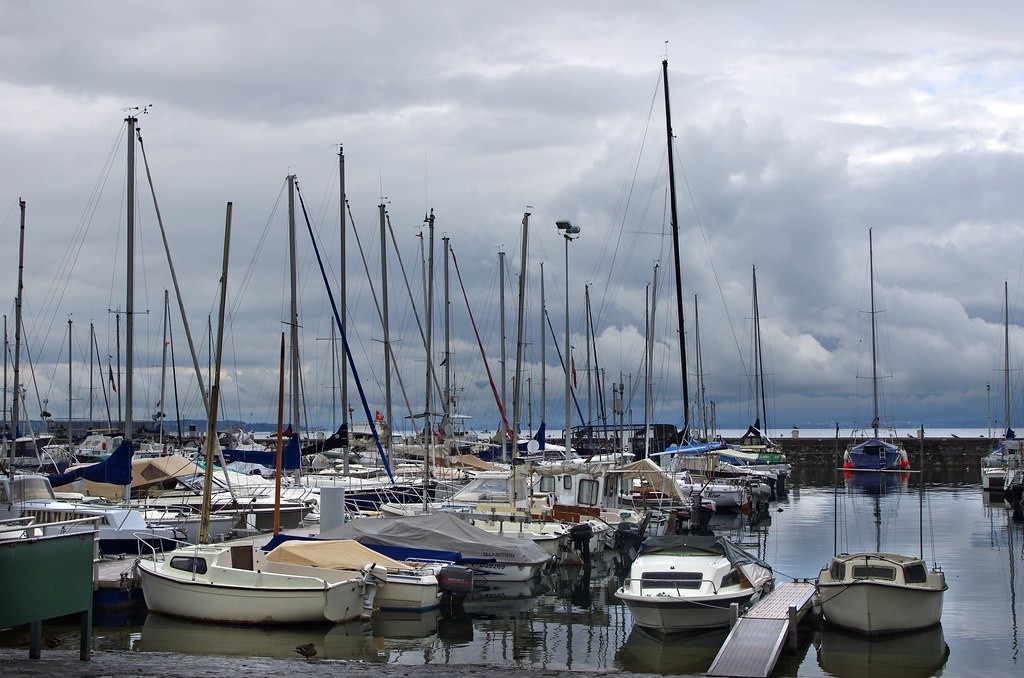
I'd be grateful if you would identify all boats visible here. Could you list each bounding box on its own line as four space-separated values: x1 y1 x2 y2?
815 422 949 638
613 534 776 635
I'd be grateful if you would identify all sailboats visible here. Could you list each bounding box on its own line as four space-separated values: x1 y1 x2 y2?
843 227 911 472
980 279 1024 492
1 40 791 626
